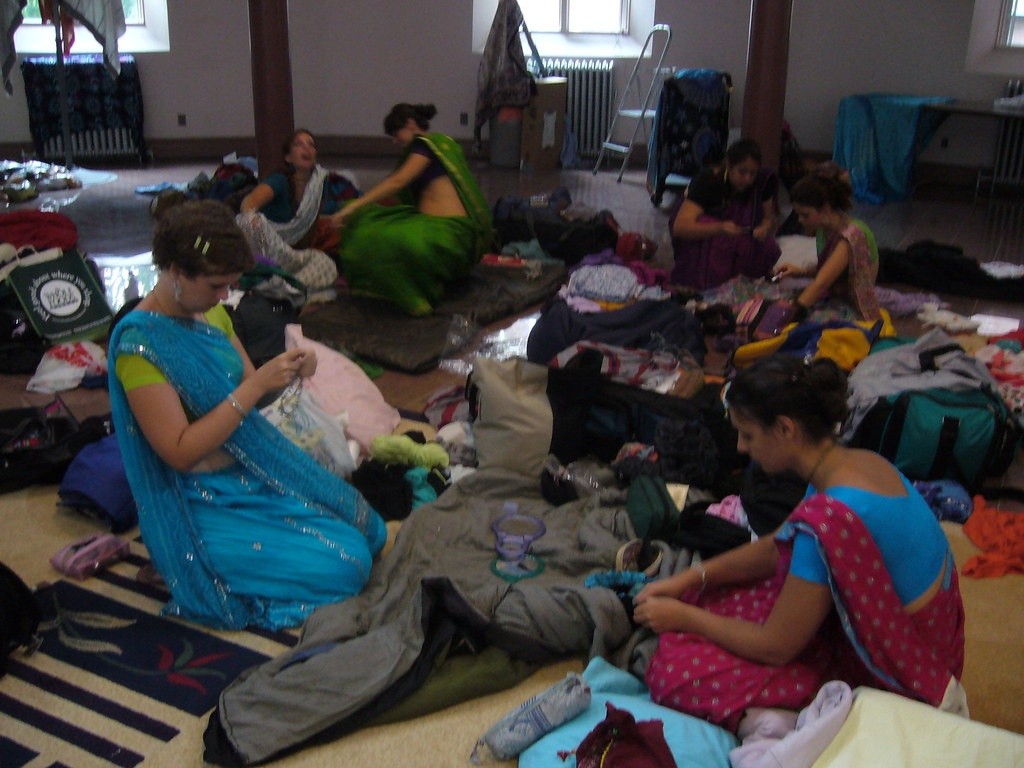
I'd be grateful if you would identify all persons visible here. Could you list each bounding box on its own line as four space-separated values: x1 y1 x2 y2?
632 355 966 734
332 103 492 319
235 129 358 302
667 138 783 292
106 197 384 634
773 164 880 320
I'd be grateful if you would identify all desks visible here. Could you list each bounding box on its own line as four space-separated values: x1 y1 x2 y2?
832 91 1024 205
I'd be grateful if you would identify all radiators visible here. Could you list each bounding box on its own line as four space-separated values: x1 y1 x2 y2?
20 54 142 162
976 78 1024 193
526 58 613 168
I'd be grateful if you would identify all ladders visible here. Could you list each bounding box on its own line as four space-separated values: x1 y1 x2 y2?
593 23 677 183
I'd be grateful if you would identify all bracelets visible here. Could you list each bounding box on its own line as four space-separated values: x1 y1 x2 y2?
227 393 249 417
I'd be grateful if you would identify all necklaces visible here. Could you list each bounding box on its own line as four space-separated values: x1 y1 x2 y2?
805 441 835 484
151 289 168 313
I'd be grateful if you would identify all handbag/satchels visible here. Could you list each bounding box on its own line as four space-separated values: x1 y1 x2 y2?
232 267 308 367
677 499 751 560
26 341 108 394
843 342 1021 500
525 290 710 366
5 244 113 346
627 476 679 544
